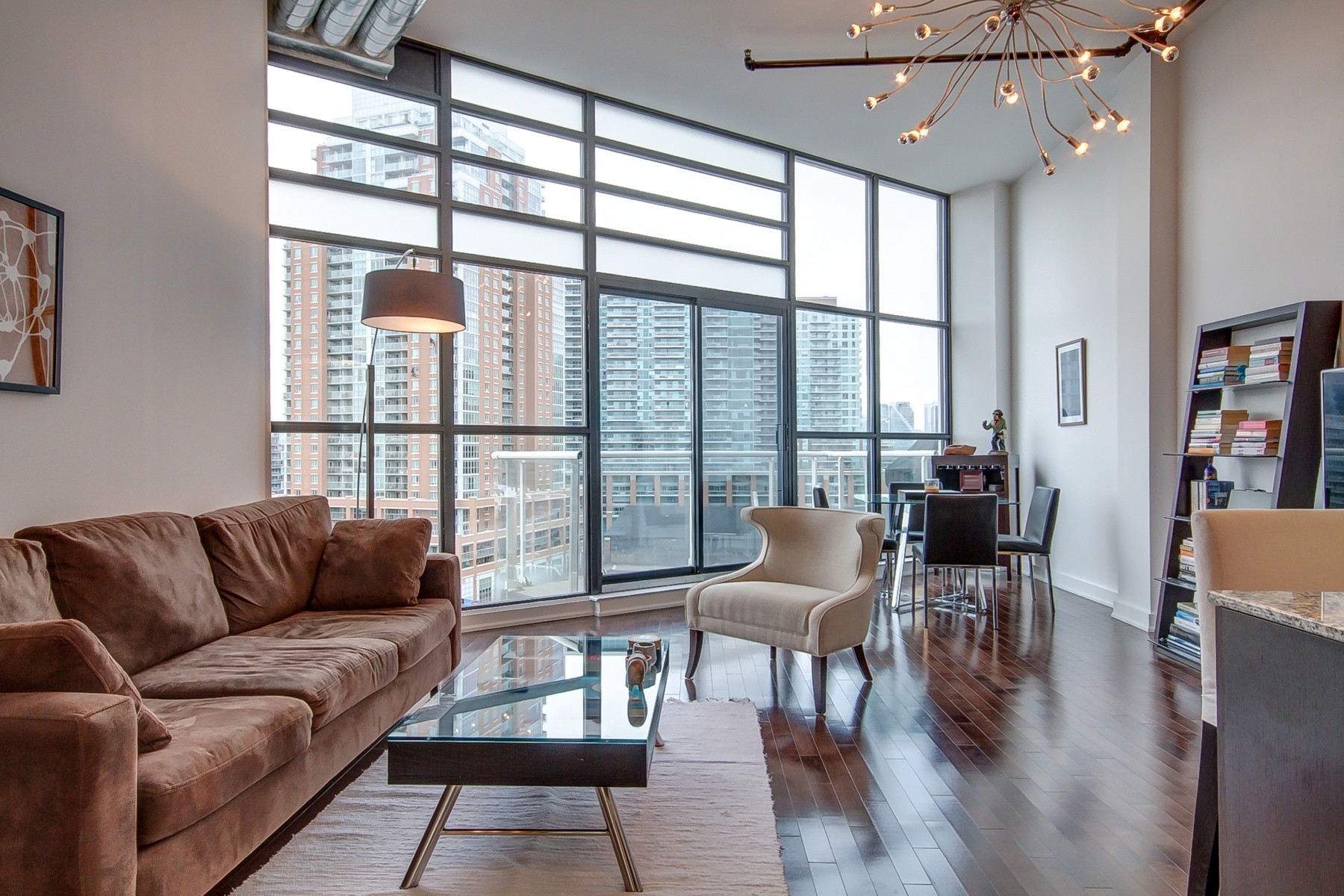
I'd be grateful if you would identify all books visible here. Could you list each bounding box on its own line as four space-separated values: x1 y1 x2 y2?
1178 537 1196 583
1167 602 1200 661
1188 410 1282 455
1191 480 1234 512
1192 336 1294 389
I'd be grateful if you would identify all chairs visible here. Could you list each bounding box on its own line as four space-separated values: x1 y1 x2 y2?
684 506 886 714
963 484 1061 612
888 481 1000 631
1186 508 1344 896
813 486 897 598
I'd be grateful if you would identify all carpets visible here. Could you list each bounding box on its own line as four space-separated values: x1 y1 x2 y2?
230 697 791 896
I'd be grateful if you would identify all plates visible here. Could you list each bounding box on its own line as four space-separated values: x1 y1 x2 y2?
989 485 1004 492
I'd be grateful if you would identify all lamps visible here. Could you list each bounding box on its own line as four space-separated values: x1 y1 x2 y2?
847 0 1186 175
355 247 468 520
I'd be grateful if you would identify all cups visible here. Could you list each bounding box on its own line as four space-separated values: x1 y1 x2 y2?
924 479 943 494
625 654 653 673
633 642 657 667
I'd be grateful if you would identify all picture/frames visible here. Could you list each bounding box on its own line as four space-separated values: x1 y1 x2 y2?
1055 338 1086 426
0 186 65 394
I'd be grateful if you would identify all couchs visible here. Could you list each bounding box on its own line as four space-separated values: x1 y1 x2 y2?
0 493 463 896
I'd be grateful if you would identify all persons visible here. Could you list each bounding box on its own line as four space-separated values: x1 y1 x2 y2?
982 409 1006 452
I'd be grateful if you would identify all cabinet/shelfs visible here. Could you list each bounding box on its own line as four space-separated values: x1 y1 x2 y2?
1145 301 1342 672
931 454 1021 581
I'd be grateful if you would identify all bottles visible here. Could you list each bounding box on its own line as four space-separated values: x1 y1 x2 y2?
1204 457 1217 480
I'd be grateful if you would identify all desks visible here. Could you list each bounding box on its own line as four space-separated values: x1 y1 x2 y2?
1205 591 1344 896
856 492 1011 606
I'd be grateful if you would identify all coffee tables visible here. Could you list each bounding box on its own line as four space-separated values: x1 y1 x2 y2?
386 636 670 891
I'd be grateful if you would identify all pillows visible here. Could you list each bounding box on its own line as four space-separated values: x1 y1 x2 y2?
0 619 173 752
309 517 432 610
